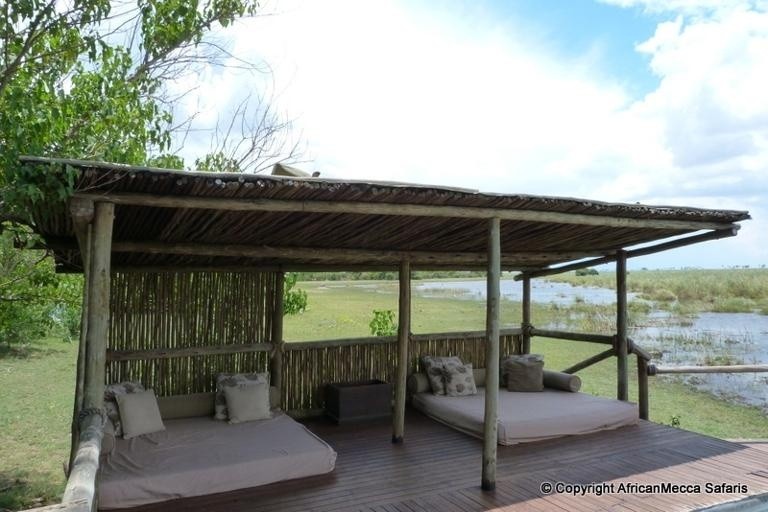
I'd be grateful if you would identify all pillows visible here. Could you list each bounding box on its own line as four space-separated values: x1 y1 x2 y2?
214 371 274 424
422 354 478 397
103 381 167 439
501 353 545 391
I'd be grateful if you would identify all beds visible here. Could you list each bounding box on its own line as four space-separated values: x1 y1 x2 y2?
96 383 336 512
408 363 639 447
